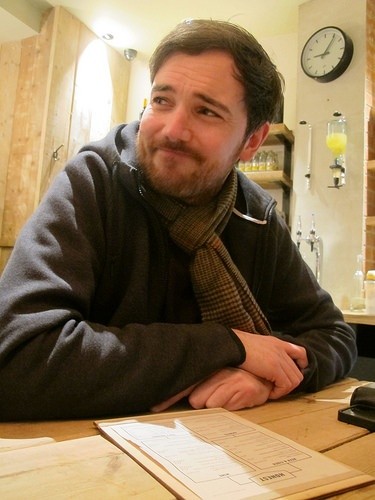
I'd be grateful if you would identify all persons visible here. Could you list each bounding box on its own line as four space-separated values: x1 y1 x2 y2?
0 18 358 414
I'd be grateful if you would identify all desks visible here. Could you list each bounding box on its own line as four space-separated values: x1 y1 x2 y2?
2 377 375 500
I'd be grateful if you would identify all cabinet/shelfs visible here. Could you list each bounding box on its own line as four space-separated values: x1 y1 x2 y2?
236 122 295 229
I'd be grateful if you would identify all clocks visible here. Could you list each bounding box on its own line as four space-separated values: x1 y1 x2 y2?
300 26 354 83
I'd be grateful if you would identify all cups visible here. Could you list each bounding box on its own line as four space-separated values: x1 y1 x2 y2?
364 270 375 315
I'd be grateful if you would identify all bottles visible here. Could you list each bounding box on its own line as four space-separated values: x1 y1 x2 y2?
348 255 365 313
235 150 279 172
